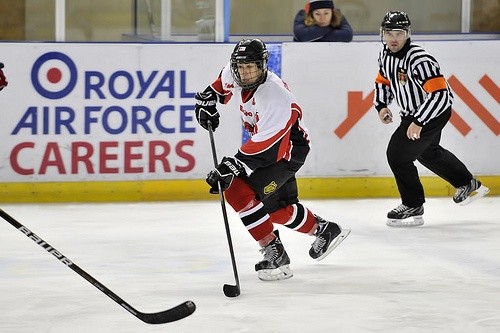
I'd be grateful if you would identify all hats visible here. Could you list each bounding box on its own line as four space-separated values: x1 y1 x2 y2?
305 0 334 18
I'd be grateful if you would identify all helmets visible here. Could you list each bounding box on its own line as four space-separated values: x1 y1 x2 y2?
228 38 270 91
380 10 412 34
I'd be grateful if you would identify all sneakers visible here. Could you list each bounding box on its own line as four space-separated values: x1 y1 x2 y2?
385 204 424 226
308 213 351 261
452 177 490 207
254 229 294 280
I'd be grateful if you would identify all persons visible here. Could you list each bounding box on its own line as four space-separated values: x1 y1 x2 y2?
373 10 481 220
195 38 342 272
293 0 352 43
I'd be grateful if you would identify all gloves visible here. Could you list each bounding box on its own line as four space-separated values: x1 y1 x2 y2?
206 155 243 194
194 92 220 132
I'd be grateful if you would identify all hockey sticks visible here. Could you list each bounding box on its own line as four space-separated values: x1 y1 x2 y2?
0 208 196 325
207 120 241 297
